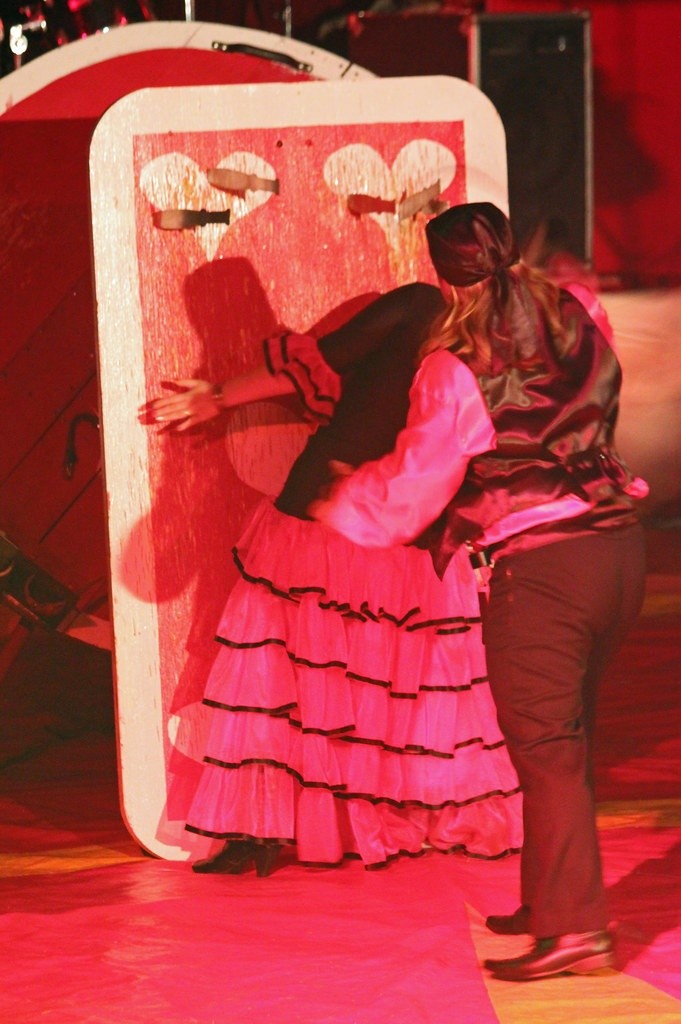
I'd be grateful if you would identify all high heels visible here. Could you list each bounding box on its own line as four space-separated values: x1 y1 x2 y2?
192 839 277 878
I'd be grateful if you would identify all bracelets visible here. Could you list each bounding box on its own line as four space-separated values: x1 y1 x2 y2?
214 381 228 408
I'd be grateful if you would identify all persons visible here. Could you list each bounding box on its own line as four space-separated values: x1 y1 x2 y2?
318 200 648 981
152 284 522 879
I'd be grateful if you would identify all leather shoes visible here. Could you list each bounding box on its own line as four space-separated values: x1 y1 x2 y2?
484 930 614 980
485 905 533 936
